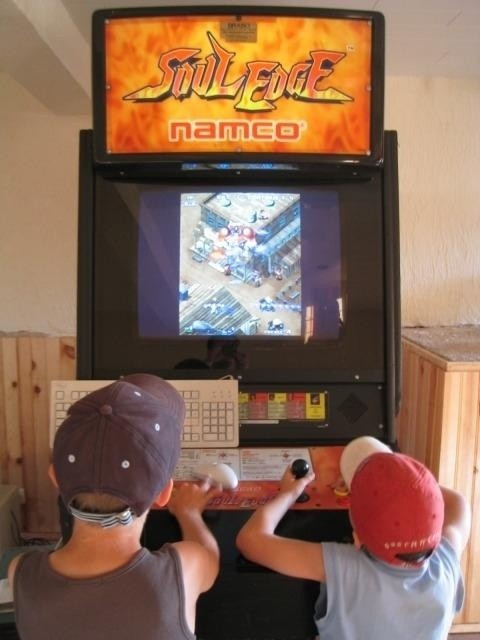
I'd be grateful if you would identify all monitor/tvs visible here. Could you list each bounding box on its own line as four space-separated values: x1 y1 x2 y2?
94 181 385 383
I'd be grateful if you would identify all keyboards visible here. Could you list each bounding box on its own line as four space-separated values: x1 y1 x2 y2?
49 379 240 448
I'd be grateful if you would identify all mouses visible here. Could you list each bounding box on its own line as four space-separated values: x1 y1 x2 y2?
193 461 239 491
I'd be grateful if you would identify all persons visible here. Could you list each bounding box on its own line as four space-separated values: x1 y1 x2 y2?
6 372 224 640
235 436 472 640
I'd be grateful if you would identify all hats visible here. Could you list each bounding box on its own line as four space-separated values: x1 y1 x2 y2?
53 372 186 526
339 436 445 569
